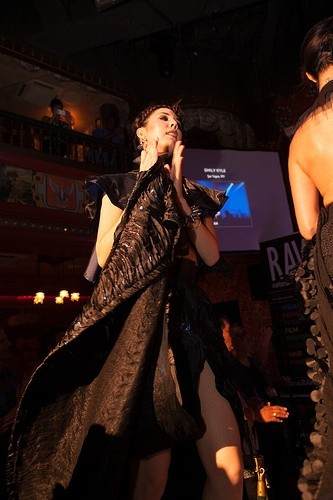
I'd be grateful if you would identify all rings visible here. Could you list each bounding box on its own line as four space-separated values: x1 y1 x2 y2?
274 413 276 417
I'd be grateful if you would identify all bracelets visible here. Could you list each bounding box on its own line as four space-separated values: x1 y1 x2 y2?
185 205 201 230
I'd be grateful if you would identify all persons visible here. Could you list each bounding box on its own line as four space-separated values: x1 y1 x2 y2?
288 17 333 500
42 100 72 156
6 98 243 500
215 316 289 500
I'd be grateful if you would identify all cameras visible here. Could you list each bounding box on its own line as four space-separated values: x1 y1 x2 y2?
56 109 65 115
